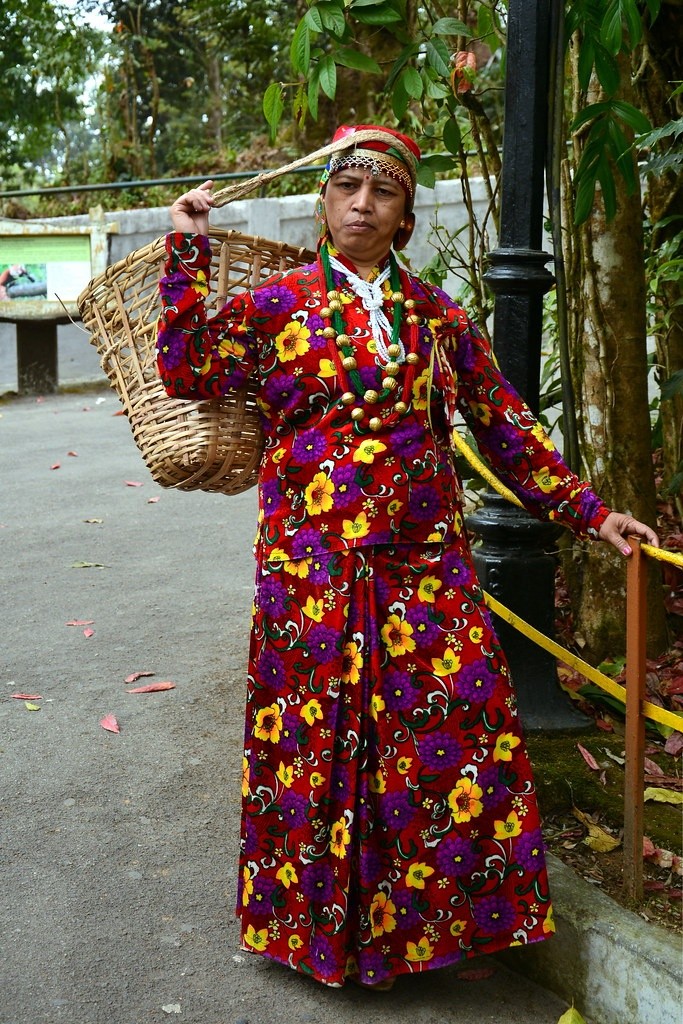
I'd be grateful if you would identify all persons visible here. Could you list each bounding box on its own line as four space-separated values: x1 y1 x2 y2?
156 126 657 995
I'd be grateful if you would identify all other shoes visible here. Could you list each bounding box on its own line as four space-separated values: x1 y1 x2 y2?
444 957 504 989
350 972 396 991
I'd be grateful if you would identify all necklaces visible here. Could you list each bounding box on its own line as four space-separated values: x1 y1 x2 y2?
311 228 423 434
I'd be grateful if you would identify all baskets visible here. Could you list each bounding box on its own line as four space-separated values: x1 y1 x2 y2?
77 226 319 496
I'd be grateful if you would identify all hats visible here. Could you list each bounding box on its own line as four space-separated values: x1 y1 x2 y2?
319 125 421 212
